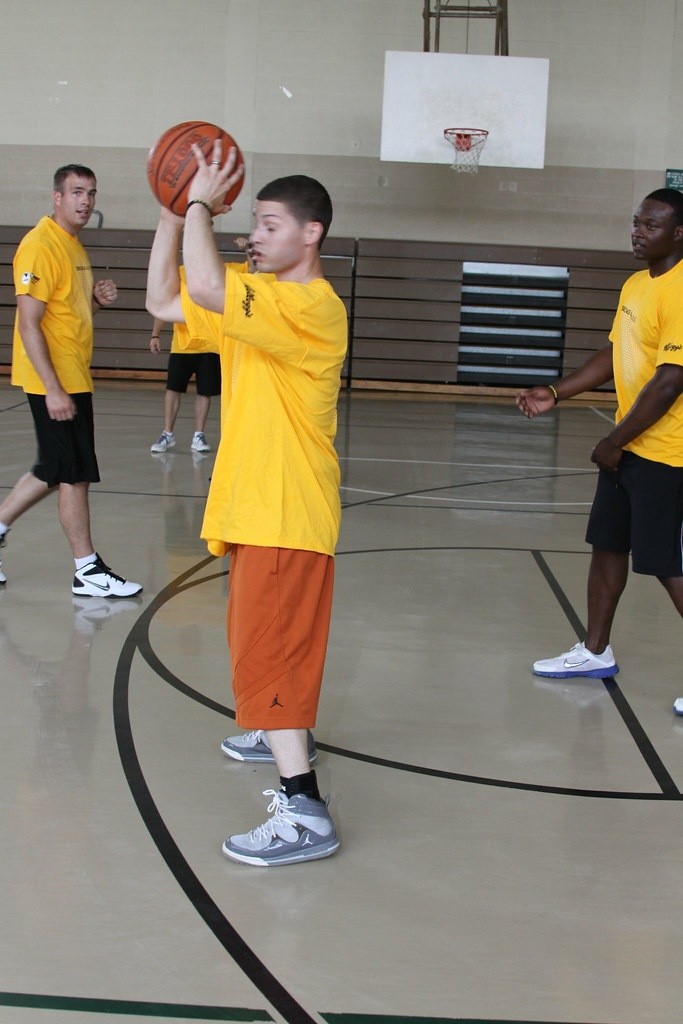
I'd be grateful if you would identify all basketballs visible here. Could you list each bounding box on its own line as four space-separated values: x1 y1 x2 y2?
145 120 246 219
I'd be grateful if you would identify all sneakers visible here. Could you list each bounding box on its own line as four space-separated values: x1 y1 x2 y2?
191 449 210 462
72 551 143 596
221 787 342 866
0 531 7 584
151 450 176 464
150 430 177 453
72 597 143 633
191 433 210 451
534 637 619 679
673 696 683 715
220 723 317 766
536 682 614 705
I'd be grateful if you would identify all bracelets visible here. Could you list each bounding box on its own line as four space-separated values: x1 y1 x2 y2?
541 384 558 405
187 199 213 212
152 336 159 338
93 286 104 306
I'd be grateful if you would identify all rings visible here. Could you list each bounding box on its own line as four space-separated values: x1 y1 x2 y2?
211 161 221 165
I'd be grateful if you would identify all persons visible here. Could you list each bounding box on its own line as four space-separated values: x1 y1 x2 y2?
150 265 222 453
145 137 349 866
515 188 683 715
0 163 144 599
